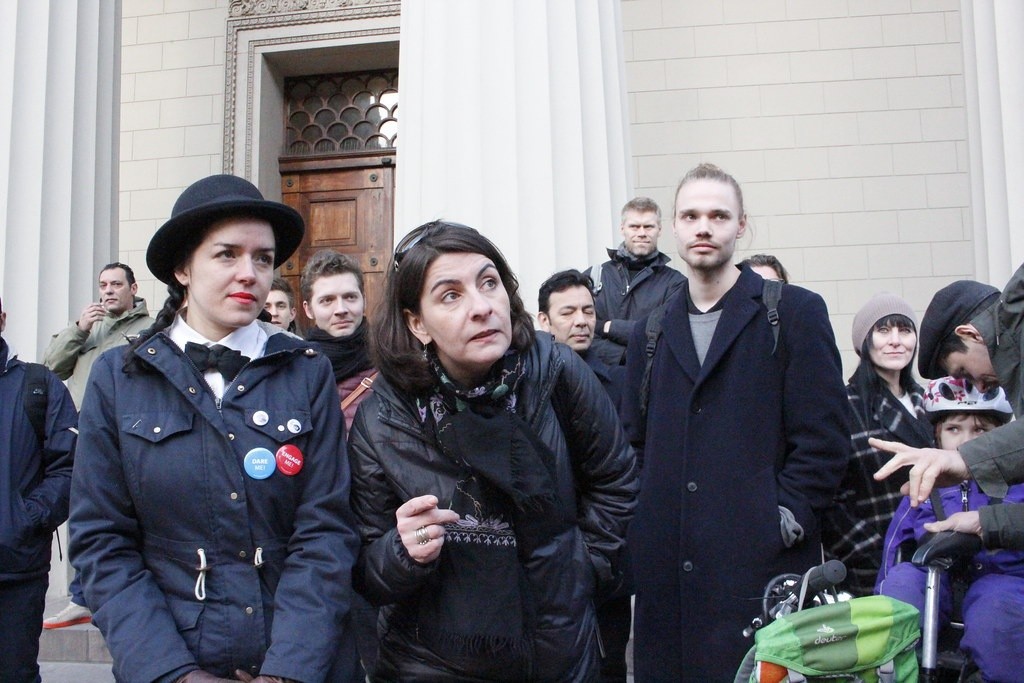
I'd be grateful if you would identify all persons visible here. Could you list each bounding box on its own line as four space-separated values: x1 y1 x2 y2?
621 162 853 583
261 248 385 683
821 265 1024 683
0 298 78 683
41 263 158 628
740 254 789 283
347 220 643 683
535 268 631 683
579 197 687 367
68 173 362 683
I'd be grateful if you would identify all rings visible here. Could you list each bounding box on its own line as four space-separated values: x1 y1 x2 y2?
416 527 432 545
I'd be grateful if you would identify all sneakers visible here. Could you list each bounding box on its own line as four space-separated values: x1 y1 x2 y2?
43 601 91 629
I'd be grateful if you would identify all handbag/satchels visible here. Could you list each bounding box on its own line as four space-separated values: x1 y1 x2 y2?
735 567 921 683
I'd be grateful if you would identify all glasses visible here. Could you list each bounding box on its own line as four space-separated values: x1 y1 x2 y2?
393 220 477 272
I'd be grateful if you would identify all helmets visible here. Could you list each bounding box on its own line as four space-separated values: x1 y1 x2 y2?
922 374 1013 423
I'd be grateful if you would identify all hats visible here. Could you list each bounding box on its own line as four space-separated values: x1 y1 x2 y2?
146 174 305 286
917 280 1002 379
851 298 919 360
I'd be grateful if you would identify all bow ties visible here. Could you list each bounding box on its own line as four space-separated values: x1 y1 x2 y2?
185 341 250 381
